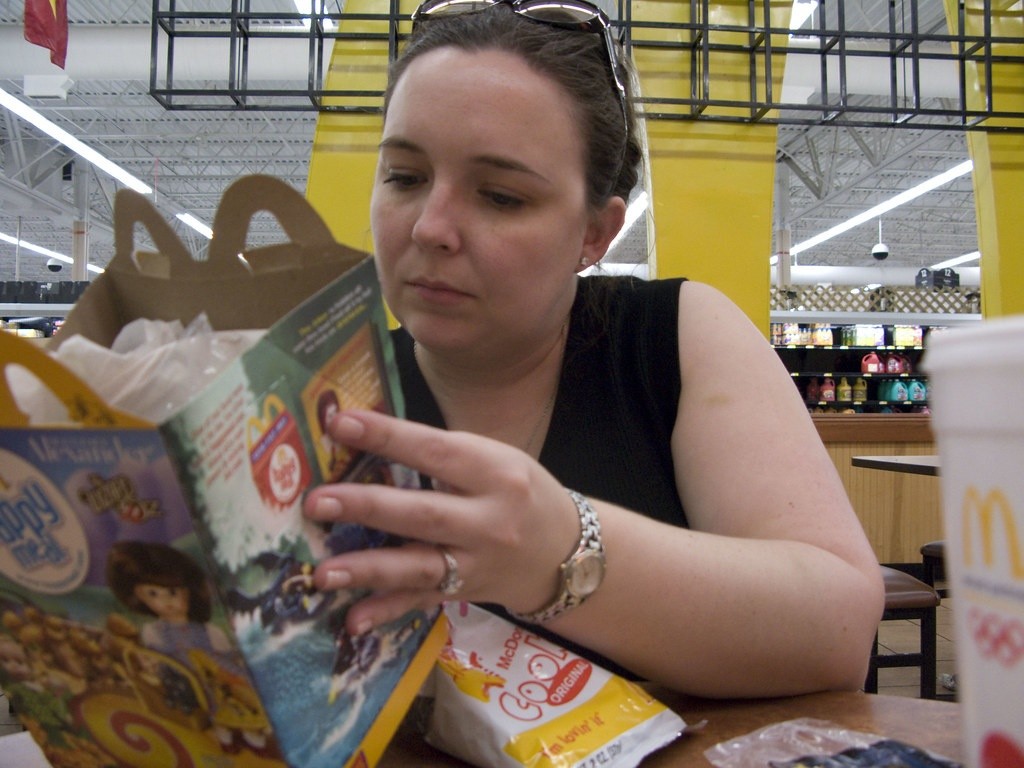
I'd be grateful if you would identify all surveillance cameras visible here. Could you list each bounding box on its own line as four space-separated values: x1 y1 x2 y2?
871 244 889 260
46 258 62 272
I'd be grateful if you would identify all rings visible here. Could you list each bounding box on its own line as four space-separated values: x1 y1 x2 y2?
433 544 463 597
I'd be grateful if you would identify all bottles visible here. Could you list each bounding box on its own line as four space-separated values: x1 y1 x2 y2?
807 378 820 401
771 323 833 346
836 377 851 401
808 405 864 413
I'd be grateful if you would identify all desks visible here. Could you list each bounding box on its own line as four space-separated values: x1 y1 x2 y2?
850 456 941 476
0 687 965 768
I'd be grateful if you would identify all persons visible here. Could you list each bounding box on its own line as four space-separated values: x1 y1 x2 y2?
302 1 886 704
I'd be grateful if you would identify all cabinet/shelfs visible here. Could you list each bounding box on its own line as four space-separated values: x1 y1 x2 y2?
0 302 78 353
770 283 983 598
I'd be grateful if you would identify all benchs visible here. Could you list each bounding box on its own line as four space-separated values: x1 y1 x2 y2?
864 564 942 702
920 541 954 598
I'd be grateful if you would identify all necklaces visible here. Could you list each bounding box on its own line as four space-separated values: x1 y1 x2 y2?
524 325 568 453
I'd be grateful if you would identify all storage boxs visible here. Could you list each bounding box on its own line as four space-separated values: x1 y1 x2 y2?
915 267 959 292
0 172 454 768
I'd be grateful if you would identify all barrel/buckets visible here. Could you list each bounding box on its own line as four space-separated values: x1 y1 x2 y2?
870 404 932 414
878 378 932 401
861 351 912 374
852 378 867 401
820 378 835 401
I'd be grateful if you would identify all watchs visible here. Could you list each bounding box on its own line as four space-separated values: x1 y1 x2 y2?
502 486 609 627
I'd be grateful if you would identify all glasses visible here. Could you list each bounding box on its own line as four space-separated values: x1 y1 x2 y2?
411 0 628 199
